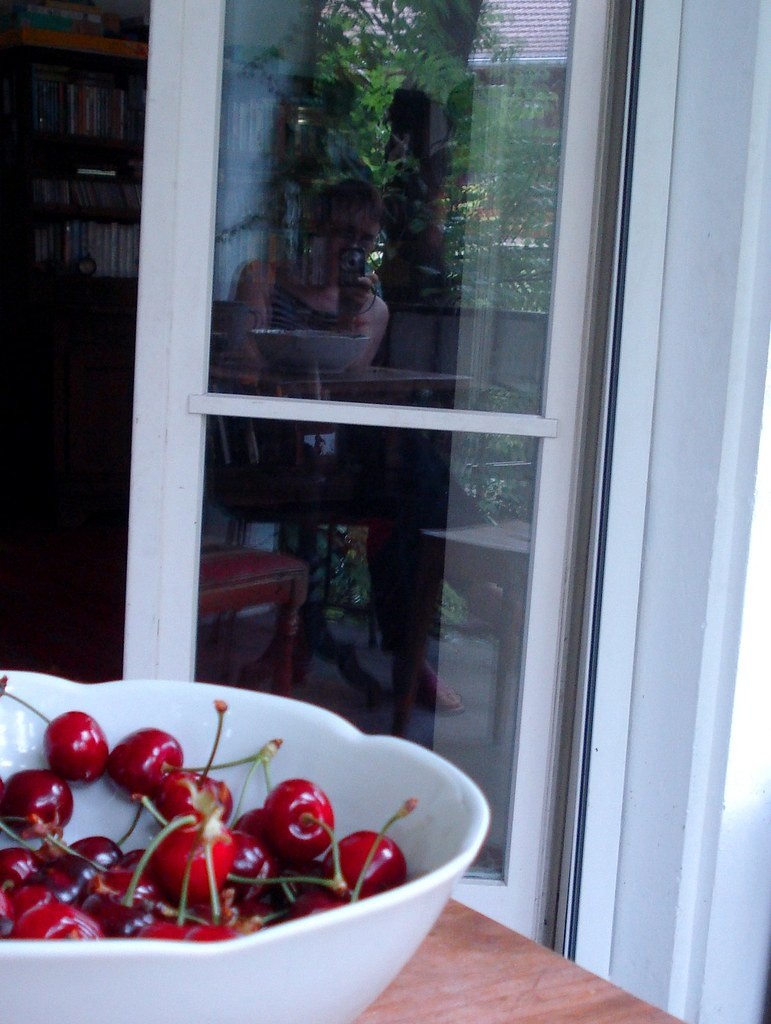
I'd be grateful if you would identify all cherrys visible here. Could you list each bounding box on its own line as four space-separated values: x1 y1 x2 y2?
0 678 417 940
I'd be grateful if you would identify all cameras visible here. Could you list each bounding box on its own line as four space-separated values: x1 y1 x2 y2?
338 246 367 288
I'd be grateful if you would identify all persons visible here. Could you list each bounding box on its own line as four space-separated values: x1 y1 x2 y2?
214 178 524 712
370 88 455 304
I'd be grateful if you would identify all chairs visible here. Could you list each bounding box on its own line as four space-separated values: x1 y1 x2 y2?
208 298 398 713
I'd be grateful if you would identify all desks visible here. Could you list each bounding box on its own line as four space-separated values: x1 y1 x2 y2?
206 352 475 740
356 898 695 1024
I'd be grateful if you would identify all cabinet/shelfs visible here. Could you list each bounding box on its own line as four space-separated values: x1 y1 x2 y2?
0 40 151 525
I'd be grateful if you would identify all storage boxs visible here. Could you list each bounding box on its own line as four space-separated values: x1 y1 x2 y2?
1 0 106 36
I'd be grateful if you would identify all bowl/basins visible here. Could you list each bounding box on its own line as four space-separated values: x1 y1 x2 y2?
252 329 371 374
1 668 492 1024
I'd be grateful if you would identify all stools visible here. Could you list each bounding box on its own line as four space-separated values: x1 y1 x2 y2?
196 544 312 699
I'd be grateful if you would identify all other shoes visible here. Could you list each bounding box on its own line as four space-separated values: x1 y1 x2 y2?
404 675 465 715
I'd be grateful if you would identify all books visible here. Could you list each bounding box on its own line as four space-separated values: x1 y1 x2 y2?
35 176 142 213
34 221 140 280
32 63 146 143
